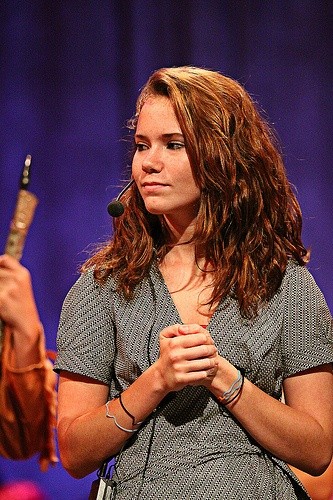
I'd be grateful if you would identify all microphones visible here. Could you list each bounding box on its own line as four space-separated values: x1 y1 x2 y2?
107 178 135 217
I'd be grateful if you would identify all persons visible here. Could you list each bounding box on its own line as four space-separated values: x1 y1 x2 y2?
49 65 332 499
1 254 51 462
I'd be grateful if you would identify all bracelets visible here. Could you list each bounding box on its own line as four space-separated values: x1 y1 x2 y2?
216 364 245 406
114 394 145 426
105 400 139 433
